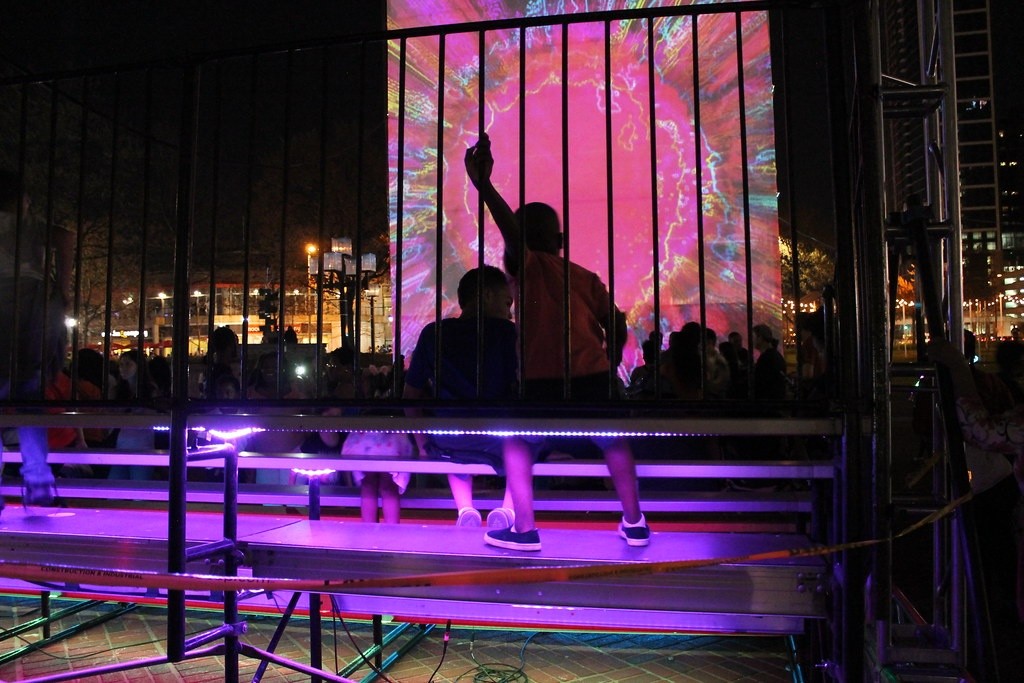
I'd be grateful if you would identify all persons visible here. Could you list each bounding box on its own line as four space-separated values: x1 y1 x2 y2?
904 328 1024 683
626 315 871 666
402 265 521 528
0 300 434 523
0 161 78 504
464 133 650 551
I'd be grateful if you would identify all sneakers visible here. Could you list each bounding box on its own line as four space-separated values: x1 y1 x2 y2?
617 513 650 546
483 524 541 551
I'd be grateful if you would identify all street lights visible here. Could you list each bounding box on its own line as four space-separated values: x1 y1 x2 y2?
194 290 203 356
317 251 376 401
899 299 908 360
157 291 167 357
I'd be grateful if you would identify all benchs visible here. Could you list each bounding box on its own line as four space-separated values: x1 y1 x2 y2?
0 400 843 683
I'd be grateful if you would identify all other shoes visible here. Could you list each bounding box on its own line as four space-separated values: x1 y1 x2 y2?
456 508 482 528
487 508 515 530
